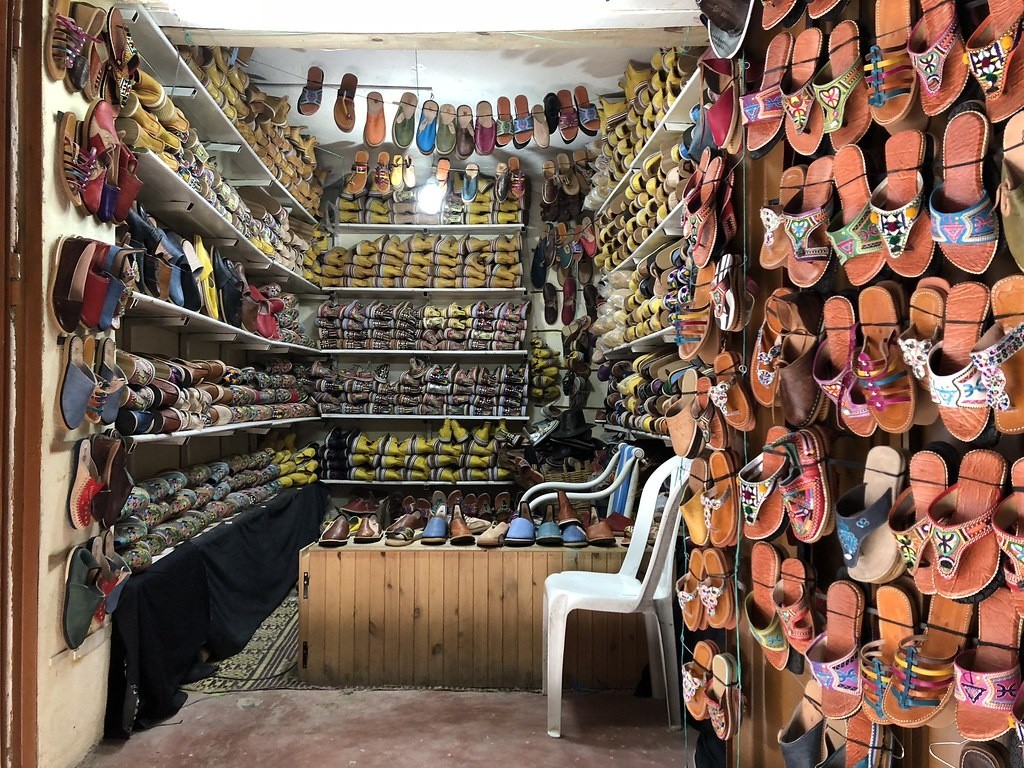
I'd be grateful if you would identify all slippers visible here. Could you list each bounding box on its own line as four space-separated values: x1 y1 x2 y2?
541 150 596 221
530 217 597 408
297 67 600 162
755 2 1024 768
665 58 738 740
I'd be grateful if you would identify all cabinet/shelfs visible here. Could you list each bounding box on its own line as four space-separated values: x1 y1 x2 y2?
113 0 530 572
597 59 731 441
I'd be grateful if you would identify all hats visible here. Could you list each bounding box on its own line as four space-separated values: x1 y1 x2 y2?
551 408 597 438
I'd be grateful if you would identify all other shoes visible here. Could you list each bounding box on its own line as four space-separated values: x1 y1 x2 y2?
46 0 131 650
337 150 526 224
593 48 688 502
115 45 335 566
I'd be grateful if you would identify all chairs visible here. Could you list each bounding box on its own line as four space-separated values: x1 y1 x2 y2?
543 455 694 737
517 443 644 519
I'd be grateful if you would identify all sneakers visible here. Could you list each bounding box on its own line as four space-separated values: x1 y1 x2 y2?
530 402 608 472
312 356 529 415
316 300 530 350
325 419 527 481
321 231 523 288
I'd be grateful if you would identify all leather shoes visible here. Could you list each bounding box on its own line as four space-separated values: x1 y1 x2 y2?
319 490 685 547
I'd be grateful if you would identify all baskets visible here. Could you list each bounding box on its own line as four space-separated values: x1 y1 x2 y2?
540 472 613 524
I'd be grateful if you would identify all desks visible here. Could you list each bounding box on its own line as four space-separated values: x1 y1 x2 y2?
298 530 652 691
